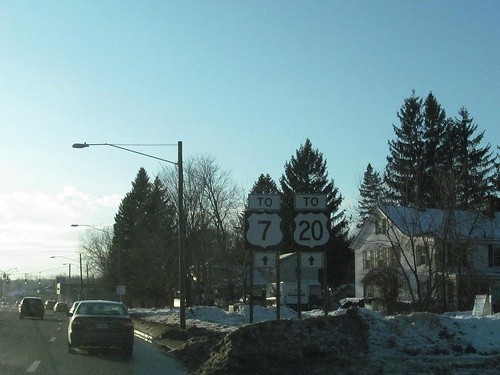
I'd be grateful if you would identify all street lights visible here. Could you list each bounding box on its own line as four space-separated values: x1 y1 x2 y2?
37 280 44 300
50 255 83 300
72 141 187 330
0 267 17 300
24 279 27 284
72 224 121 302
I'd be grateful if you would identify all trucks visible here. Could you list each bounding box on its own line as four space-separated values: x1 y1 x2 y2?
273 280 309 311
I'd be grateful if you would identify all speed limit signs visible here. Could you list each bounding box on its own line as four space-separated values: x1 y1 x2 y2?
294 193 329 269
247 193 283 269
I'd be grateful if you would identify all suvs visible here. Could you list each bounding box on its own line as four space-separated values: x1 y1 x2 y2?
20 297 45 320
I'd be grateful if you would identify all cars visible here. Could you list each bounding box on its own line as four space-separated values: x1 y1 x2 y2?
15 301 20 308
45 301 56 311
67 301 79 320
248 288 264 301
2 299 10 306
67 300 134 355
52 302 68 313
